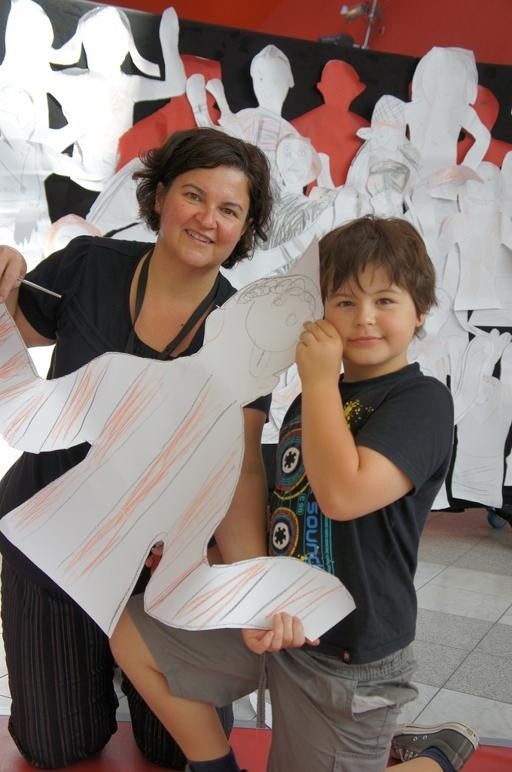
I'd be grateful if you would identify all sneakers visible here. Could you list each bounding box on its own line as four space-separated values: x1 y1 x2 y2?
390 722 478 772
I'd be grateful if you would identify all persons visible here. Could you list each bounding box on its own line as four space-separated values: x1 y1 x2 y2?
107 214 480 772
0 129 322 767
